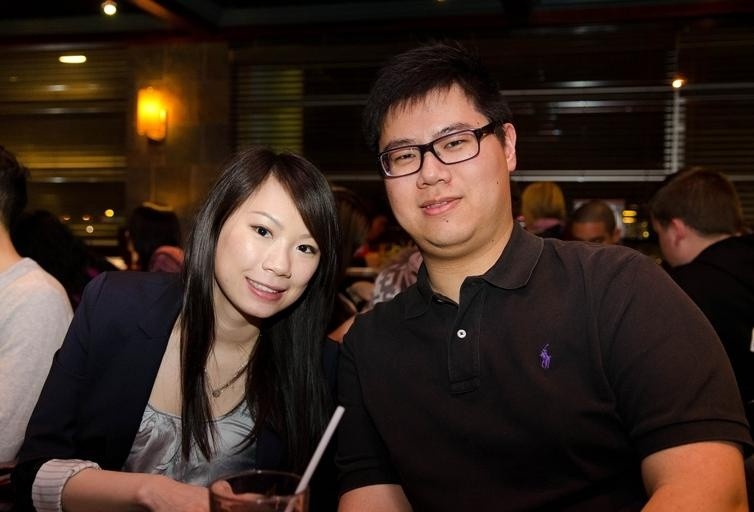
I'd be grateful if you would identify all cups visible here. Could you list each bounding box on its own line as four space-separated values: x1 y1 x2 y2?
209 470 311 512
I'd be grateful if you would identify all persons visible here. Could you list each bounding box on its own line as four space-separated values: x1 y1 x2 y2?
1 43 753 511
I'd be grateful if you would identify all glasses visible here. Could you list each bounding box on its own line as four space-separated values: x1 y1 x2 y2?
374 122 496 180
